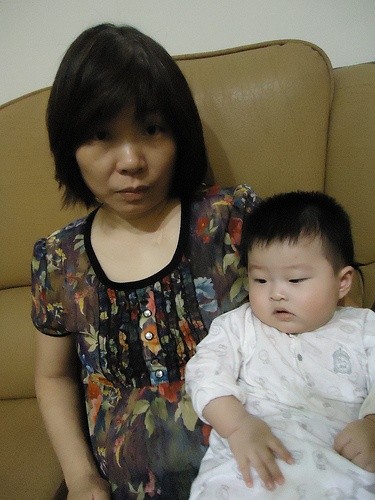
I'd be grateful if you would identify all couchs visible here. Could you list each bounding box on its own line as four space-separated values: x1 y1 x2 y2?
0 39 375 500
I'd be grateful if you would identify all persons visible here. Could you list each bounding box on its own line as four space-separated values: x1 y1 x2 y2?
29 21 272 500
185 190 375 500
